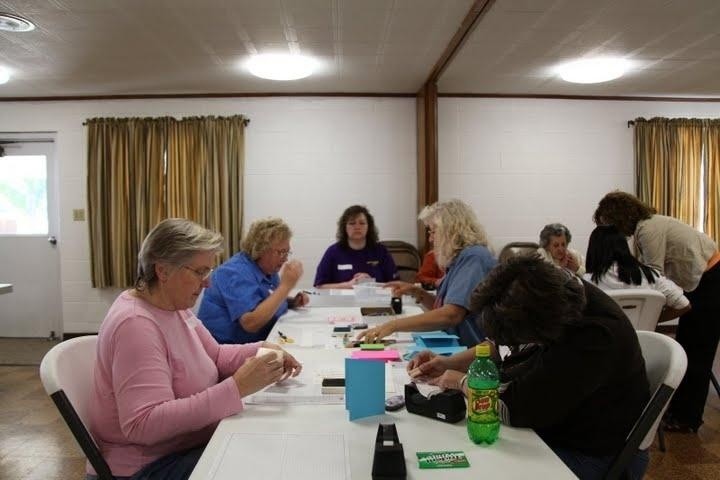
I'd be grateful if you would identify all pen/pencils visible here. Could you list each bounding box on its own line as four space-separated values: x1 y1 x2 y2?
303 290 321 295
278 331 287 339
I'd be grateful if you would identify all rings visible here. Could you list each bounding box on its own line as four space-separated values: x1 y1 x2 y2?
372 332 379 337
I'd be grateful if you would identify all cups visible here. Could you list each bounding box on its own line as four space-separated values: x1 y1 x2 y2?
256 347 283 382
363 278 377 292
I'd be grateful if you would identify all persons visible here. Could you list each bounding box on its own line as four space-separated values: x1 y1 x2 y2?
312 204 400 289
405 250 649 479
593 190 720 436
83 216 303 480
535 223 587 279
416 248 450 289
582 225 692 315
196 216 310 346
355 197 500 349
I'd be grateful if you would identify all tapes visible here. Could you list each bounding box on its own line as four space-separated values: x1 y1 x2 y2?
383 441 396 446
427 390 441 400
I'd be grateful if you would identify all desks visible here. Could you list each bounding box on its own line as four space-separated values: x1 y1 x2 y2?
180 287 577 480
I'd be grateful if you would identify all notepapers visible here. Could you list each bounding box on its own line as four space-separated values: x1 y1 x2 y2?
360 344 384 351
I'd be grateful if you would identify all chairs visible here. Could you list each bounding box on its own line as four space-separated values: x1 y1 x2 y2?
39 328 114 479
380 240 420 279
607 289 667 330
498 240 539 264
602 329 690 478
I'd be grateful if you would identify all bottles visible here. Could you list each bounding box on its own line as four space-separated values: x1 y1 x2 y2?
391 297 401 313
465 343 501 444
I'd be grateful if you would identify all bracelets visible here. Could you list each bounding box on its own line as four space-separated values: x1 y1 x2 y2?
459 372 468 391
